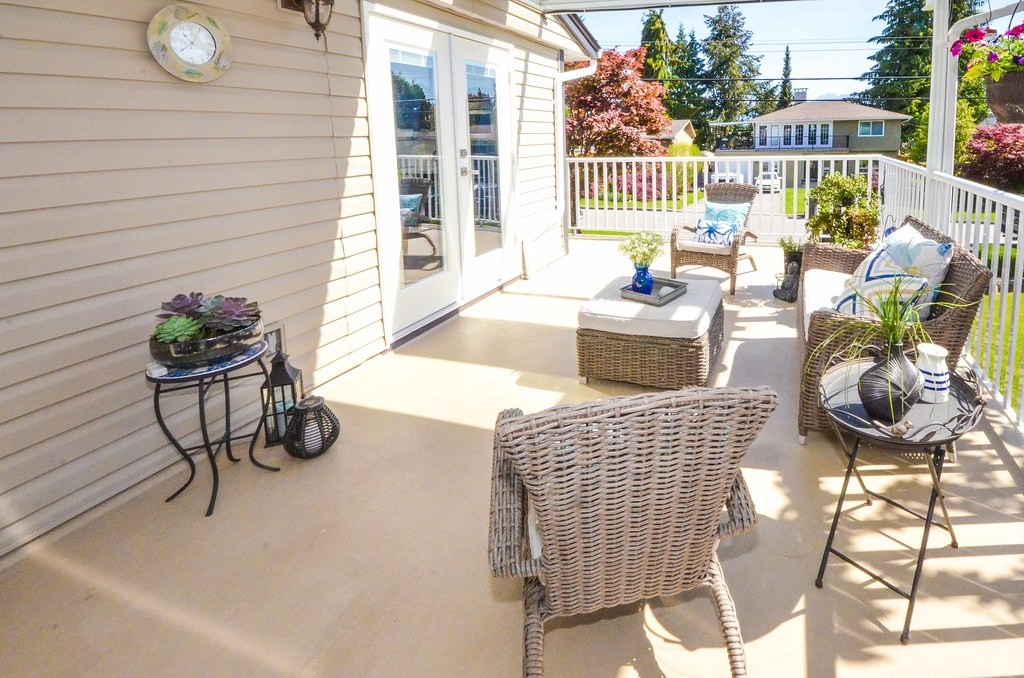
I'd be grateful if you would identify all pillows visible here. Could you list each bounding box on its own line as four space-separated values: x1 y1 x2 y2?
832 222 954 323
696 218 738 245
400 194 423 226
704 201 750 228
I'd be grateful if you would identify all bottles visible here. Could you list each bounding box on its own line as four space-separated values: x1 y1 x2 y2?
915 342 950 403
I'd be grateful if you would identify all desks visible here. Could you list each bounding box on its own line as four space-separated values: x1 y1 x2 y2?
144 341 282 517
575 275 724 390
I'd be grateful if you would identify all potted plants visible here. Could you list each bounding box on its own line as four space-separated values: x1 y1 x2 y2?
779 233 803 274
148 291 264 369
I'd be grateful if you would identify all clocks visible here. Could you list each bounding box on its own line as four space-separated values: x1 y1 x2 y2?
146 3 233 83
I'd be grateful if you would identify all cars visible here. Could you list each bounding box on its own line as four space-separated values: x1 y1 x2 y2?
754 172 782 193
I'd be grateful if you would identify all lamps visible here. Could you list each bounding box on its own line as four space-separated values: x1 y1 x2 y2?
282 395 341 458
276 0 335 41
259 340 303 449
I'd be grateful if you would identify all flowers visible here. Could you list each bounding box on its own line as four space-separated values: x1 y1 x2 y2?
951 21 1024 82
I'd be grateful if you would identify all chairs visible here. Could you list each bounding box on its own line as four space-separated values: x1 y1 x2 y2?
668 182 759 295
487 384 780 678
399 177 438 257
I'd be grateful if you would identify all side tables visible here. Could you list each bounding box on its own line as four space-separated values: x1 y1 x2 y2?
815 355 987 646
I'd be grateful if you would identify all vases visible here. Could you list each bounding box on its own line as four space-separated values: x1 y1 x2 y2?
633 263 654 294
982 72 1024 126
856 341 927 424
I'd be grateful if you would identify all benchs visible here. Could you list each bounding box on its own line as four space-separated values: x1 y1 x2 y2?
796 215 994 446
